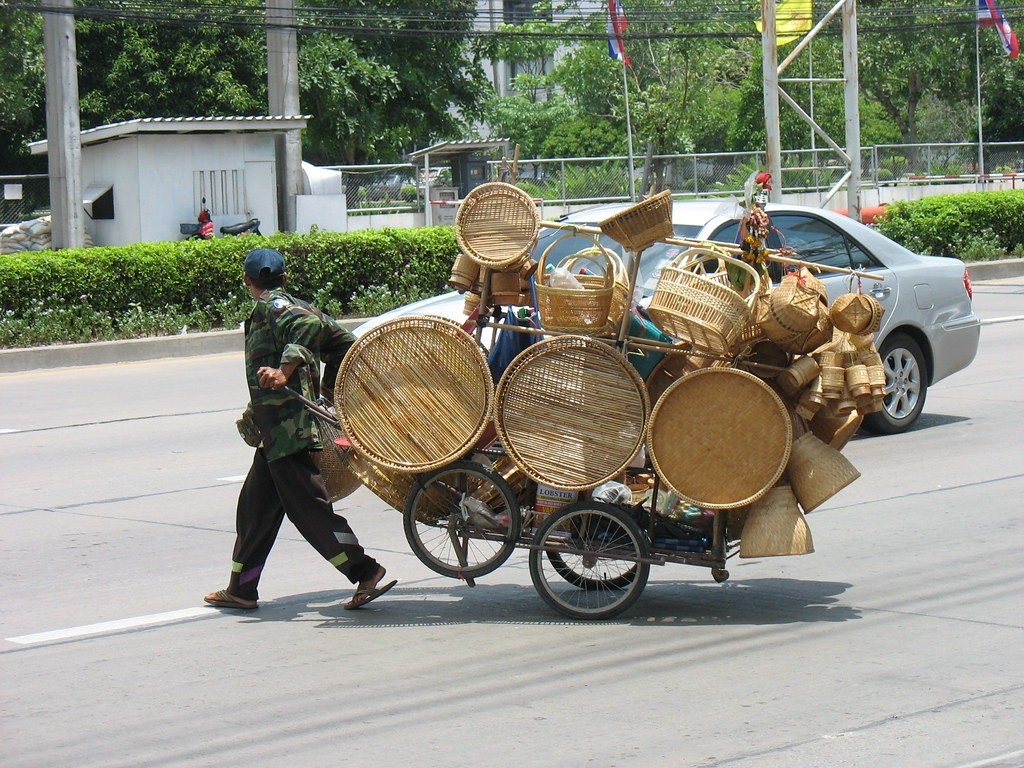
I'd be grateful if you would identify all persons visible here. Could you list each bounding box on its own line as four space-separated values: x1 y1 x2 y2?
205 246 388 611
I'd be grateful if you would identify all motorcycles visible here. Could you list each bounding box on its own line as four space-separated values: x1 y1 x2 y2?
180 197 262 240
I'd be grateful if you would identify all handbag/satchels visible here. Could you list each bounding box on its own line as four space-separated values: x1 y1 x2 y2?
235 401 262 447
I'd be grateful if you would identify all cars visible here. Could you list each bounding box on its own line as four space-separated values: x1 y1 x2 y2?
344 199 983 435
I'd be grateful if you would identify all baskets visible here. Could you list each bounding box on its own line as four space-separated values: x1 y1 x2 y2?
310 185 886 559
180 224 201 235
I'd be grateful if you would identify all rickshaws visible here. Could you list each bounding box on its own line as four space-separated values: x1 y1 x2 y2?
253 368 729 621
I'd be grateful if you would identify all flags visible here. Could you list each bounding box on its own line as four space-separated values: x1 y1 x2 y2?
976 0 1019 58
753 0 813 46
607 0 632 71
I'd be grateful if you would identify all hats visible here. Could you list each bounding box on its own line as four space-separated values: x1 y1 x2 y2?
244 250 285 277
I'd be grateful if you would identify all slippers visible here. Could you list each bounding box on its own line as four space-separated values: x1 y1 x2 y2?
344 579 399 611
204 590 259 611
